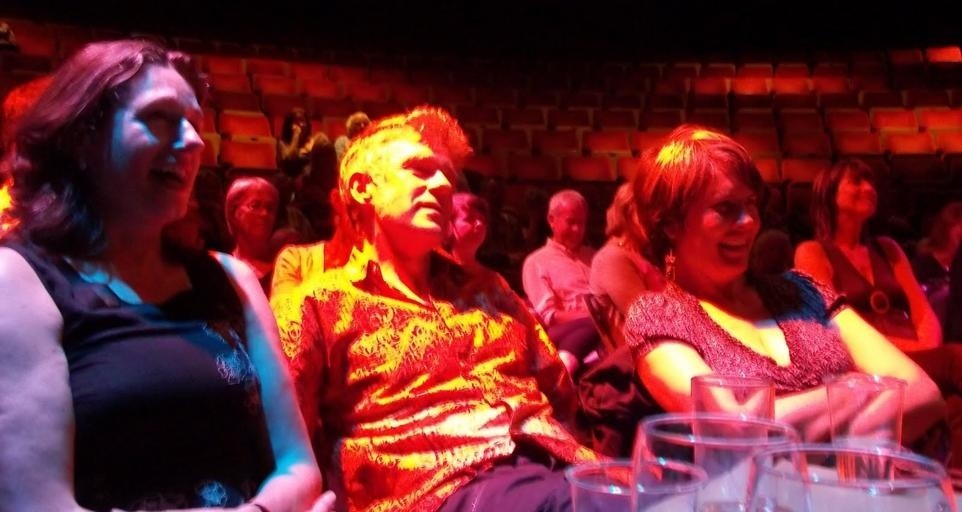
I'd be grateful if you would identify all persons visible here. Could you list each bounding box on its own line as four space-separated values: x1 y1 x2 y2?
2 38 962 512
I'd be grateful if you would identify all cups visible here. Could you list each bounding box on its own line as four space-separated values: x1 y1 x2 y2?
826 376 908 483
745 447 960 511
632 414 807 511
693 376 778 509
564 458 707 510
